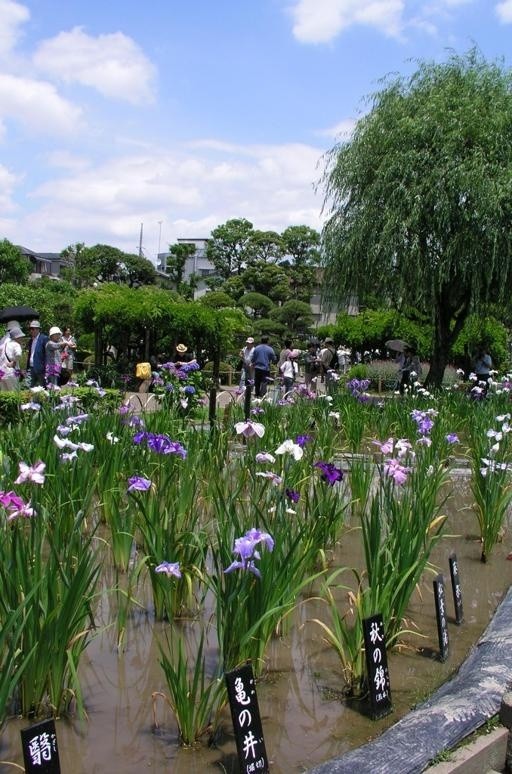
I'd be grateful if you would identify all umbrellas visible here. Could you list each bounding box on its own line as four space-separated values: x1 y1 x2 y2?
0 305 40 324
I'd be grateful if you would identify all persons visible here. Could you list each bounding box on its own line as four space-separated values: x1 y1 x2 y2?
239 335 422 396
0 320 78 392
171 344 197 366
473 345 493 390
150 355 163 378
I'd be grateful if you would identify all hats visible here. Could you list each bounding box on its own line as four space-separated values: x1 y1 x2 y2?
6 321 24 330
288 351 297 358
245 337 255 344
9 327 25 338
49 327 63 337
30 320 43 329
176 344 188 353
323 337 335 343
